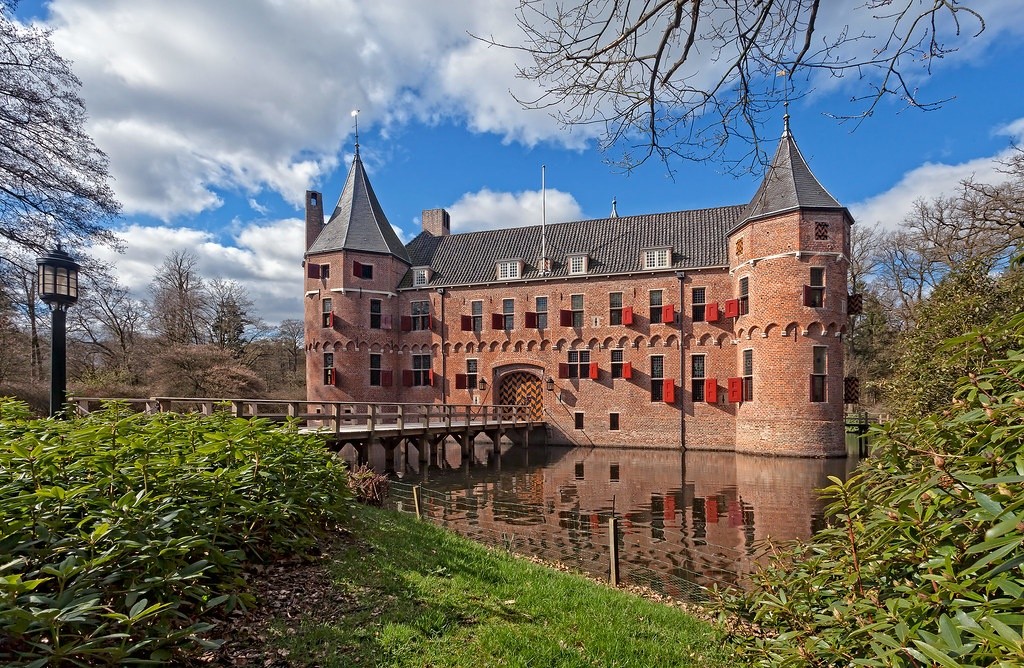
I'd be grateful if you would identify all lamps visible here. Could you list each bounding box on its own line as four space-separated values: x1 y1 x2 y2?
745 335 750 340
835 332 840 337
305 337 736 357
737 338 741 342
802 330 808 336
479 377 490 390
781 331 786 336
546 376 557 391
761 333 767 337
821 331 828 336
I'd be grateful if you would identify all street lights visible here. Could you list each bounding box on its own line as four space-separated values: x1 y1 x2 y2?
35 242 81 443
